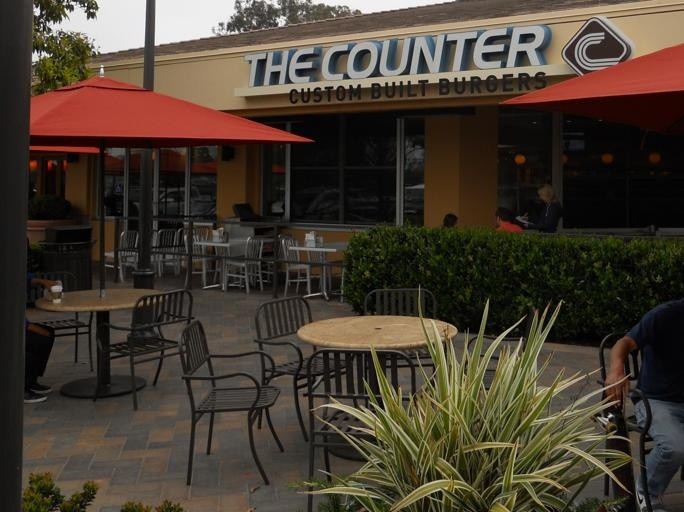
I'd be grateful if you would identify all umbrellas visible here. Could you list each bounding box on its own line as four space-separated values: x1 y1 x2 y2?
498 44 684 137
29 75 316 398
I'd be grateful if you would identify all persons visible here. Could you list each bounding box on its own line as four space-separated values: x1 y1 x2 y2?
23 272 58 403
524 183 562 232
604 298 684 512
443 214 458 227
495 207 525 233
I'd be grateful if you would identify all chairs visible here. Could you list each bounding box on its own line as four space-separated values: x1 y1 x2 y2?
305 232 333 294
253 297 349 443
282 238 324 295
35 271 93 371
93 289 194 410
225 236 265 291
592 333 684 512
117 225 224 290
464 336 526 391
178 319 284 486
307 348 414 512
364 289 439 403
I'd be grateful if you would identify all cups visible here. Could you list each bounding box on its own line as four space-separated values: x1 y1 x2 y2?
51 285 64 305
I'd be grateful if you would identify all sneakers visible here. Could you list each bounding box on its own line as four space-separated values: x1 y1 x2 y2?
24 390 48 403
29 383 52 394
636 489 667 512
